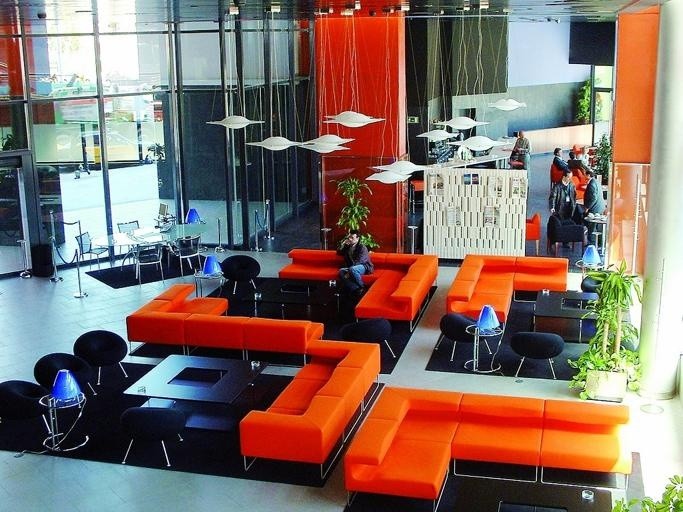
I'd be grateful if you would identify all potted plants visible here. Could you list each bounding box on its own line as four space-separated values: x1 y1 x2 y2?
327 176 381 253
567 257 644 403
576 80 603 125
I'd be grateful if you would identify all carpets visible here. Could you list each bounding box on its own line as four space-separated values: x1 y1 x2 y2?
85 255 220 289
342 452 648 512
127 277 438 375
424 288 633 381
1 361 385 486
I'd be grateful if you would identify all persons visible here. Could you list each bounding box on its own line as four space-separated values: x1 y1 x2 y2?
584 171 605 247
548 171 577 251
553 147 569 174
578 146 589 165
510 130 530 188
566 152 594 178
337 230 373 296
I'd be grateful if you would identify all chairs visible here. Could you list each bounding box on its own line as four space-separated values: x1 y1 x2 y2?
166 235 202 277
131 243 164 287
512 334 565 380
73 331 129 386
76 233 112 271
336 318 396 361
579 271 604 306
119 409 192 468
117 220 149 272
435 314 492 360
38 351 98 401
220 256 260 294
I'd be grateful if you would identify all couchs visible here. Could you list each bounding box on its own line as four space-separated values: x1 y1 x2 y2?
126 284 325 355
279 248 440 336
344 387 632 510
240 340 379 478
443 254 569 333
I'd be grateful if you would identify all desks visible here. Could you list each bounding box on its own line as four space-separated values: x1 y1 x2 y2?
463 323 503 373
123 355 268 428
193 271 224 296
245 282 342 321
38 394 90 450
533 288 601 342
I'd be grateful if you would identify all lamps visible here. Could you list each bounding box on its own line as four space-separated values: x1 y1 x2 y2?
446 2 506 153
416 8 459 141
205 1 265 129
488 9 527 111
245 1 313 151
303 3 355 147
324 3 386 128
324 3 372 123
297 7 350 155
430 7 492 131
368 5 427 174
365 3 411 186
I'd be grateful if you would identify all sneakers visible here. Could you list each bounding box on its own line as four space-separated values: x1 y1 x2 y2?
563 244 571 249
